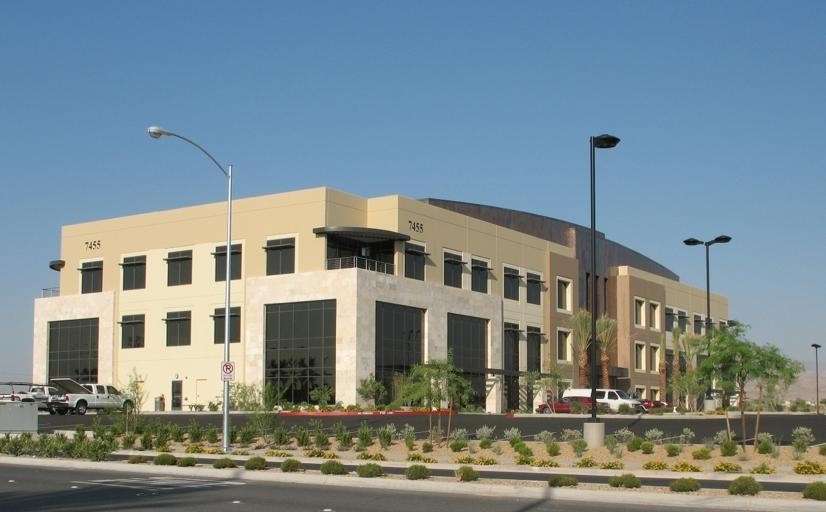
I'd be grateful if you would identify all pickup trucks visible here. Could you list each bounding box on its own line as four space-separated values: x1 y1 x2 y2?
627 390 661 413
0 376 138 416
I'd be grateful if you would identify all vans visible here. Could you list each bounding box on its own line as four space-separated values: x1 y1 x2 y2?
561 387 643 415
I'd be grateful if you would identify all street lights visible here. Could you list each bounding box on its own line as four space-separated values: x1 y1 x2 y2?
146 123 235 453
810 343 823 412
682 234 733 399
587 131 622 421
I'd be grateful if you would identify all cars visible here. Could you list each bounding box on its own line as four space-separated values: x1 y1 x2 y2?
533 395 610 413
